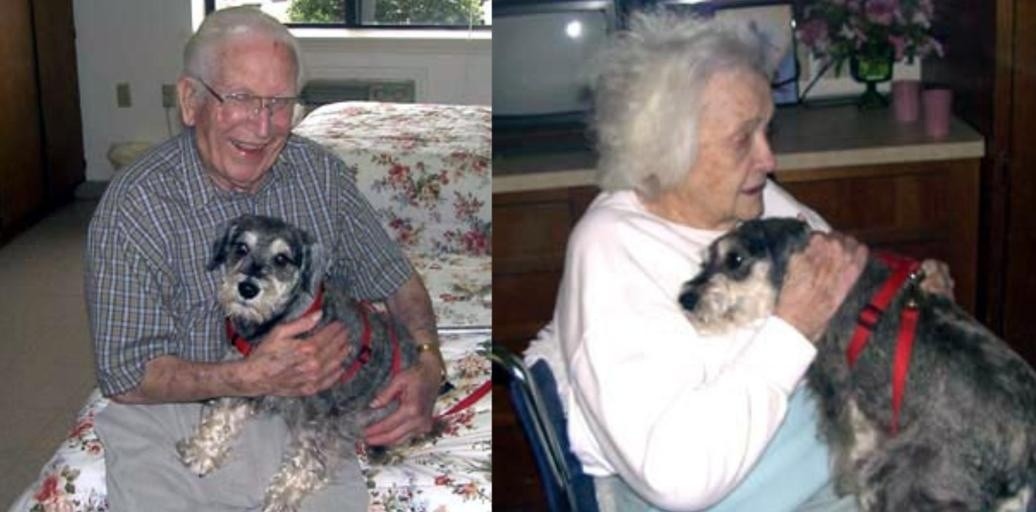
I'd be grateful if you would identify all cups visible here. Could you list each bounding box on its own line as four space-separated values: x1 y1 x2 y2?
891 79 954 135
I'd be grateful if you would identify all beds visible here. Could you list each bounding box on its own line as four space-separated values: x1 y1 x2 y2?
7 99 492 511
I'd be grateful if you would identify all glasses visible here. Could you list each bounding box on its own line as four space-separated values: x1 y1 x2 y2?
200 78 295 122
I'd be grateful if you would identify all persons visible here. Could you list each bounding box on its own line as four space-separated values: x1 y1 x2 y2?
553 10 954 511
84 5 444 511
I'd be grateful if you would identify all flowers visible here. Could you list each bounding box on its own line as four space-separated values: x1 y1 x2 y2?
795 0 946 79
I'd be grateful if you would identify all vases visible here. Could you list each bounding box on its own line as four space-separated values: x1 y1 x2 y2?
849 46 893 111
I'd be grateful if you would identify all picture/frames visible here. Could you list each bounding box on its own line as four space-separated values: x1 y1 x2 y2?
711 0 799 107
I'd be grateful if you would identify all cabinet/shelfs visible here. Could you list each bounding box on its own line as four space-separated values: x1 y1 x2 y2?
1 0 86 249
492 98 982 512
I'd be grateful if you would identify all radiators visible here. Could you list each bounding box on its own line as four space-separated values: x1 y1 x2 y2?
297 66 427 115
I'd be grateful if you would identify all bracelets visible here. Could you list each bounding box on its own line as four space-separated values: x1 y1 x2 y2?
410 344 447 385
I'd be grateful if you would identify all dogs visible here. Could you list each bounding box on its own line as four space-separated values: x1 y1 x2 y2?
176 213 455 512
677 218 1036 512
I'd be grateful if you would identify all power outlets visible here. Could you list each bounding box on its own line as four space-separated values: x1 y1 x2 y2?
162 84 177 108
